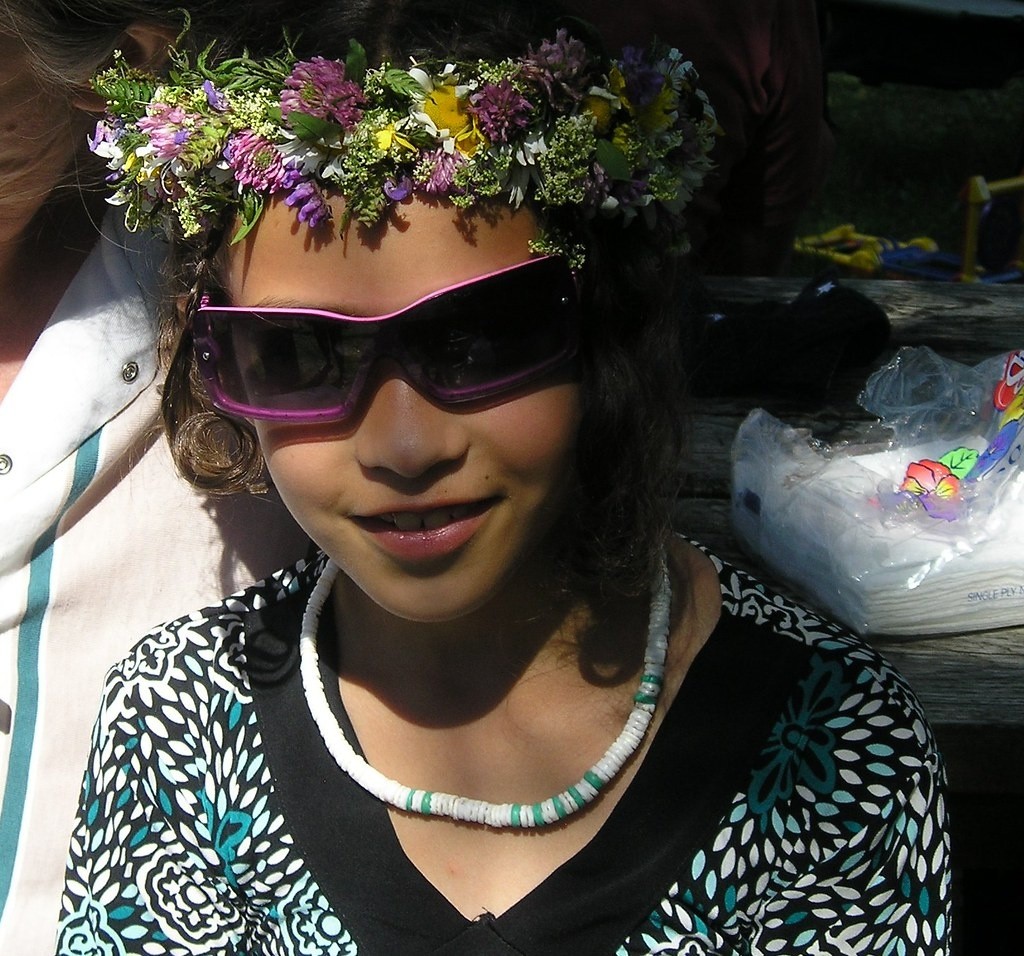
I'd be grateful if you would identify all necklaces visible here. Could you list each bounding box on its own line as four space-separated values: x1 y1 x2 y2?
299 545 671 828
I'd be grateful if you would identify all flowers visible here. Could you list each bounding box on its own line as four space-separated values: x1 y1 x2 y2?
86 28 714 309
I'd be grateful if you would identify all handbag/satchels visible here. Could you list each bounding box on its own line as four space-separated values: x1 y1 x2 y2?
729 344 1024 639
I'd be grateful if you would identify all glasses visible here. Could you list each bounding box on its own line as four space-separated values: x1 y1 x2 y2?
192 248 594 425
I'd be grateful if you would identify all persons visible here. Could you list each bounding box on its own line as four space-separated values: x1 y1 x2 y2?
1 0 952 956
